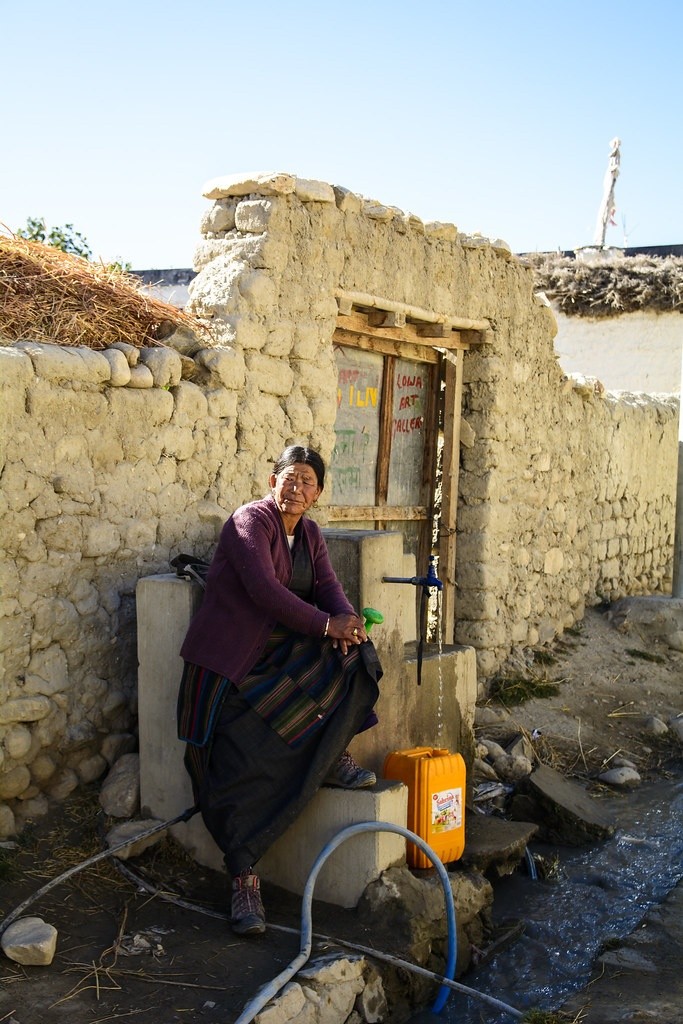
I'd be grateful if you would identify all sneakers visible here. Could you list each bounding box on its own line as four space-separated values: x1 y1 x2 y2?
229 876 266 934
324 749 377 789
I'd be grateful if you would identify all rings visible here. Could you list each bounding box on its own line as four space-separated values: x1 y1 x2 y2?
353 628 358 635
353 636 358 639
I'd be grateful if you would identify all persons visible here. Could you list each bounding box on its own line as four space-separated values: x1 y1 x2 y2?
175 444 384 937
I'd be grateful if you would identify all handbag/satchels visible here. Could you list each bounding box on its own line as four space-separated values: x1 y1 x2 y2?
171 554 210 590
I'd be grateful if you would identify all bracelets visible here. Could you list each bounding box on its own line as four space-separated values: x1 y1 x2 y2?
323 618 330 637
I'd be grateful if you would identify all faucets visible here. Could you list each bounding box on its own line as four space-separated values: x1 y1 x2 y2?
382 555 445 591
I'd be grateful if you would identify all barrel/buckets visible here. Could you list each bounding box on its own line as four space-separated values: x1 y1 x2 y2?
384 746 466 867
